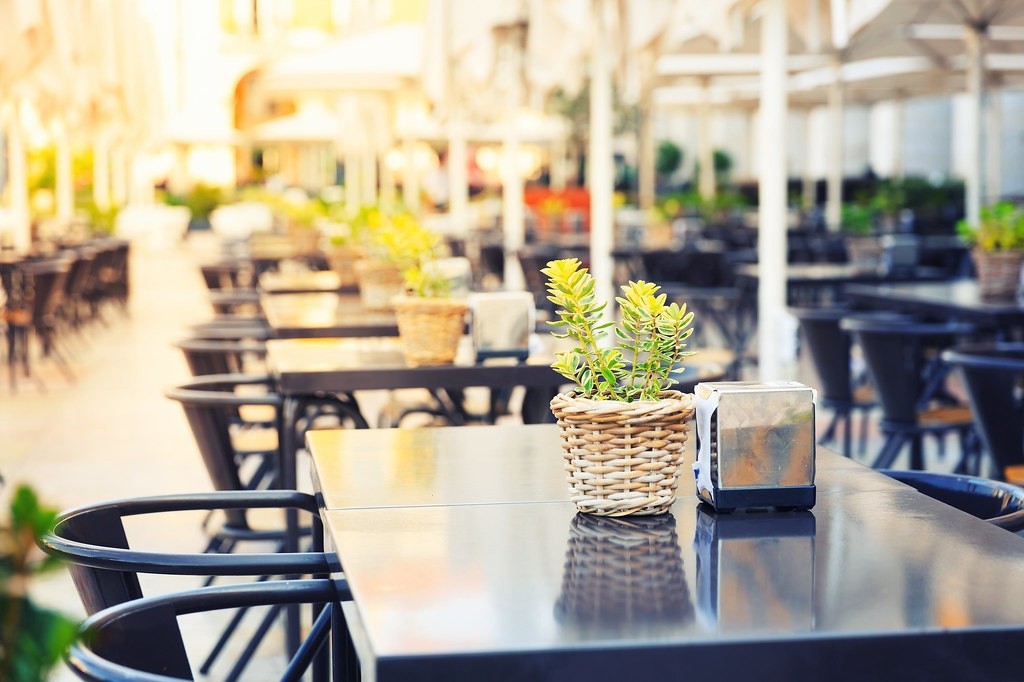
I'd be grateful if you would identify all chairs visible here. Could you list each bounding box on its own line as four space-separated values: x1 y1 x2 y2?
0 242 132 375
37 211 1024 682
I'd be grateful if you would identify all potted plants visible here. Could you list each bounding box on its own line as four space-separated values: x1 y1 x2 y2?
539 258 697 519
382 205 466 366
350 206 406 313
321 209 363 292
956 197 1024 297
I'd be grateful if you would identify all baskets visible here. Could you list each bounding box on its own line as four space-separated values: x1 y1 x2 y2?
550 388 696 516
972 249 1023 296
393 296 469 365
553 511 695 639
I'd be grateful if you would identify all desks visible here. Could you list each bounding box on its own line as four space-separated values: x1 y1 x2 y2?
261 293 469 340
305 423 917 682
847 281 1024 345
743 265 870 305
316 489 1024 682
266 336 649 663
259 269 360 297
